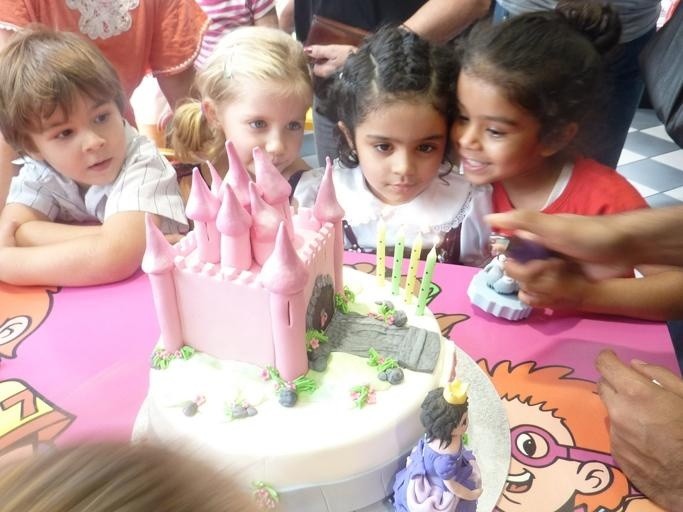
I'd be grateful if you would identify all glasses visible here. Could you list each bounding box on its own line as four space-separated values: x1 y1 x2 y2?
510 425 612 467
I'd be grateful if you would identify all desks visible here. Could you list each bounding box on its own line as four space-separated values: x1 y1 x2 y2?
1 250 682 512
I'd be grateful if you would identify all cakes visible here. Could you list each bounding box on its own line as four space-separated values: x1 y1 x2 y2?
128 141 512 512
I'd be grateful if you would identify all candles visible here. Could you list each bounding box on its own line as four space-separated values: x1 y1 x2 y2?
376 226 440 316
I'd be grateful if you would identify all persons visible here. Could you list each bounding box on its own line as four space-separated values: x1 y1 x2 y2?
479 204 682 512
0 430 259 510
